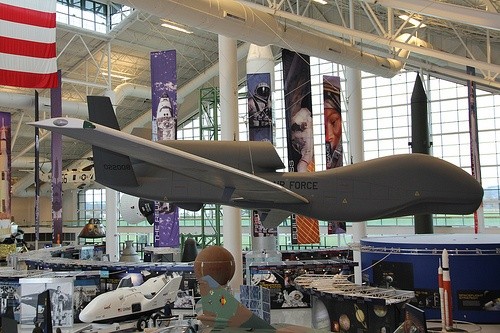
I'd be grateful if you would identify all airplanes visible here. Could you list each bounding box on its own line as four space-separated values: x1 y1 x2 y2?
78 271 183 332
24 94 485 223
